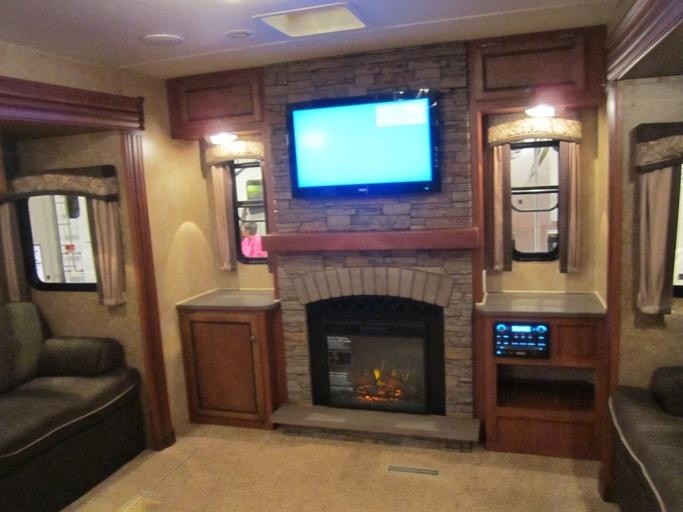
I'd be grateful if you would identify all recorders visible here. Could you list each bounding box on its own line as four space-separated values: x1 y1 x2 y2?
493 322 549 359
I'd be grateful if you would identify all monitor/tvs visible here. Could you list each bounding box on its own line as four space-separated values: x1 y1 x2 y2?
285 95 439 197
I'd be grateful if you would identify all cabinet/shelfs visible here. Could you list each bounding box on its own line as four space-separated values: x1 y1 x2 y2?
178 287 286 433
480 289 608 459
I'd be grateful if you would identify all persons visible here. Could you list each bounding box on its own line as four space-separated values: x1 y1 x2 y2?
240 222 269 257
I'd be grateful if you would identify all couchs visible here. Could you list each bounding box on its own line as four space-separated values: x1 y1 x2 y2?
0 301 142 512
599 365 683 512
